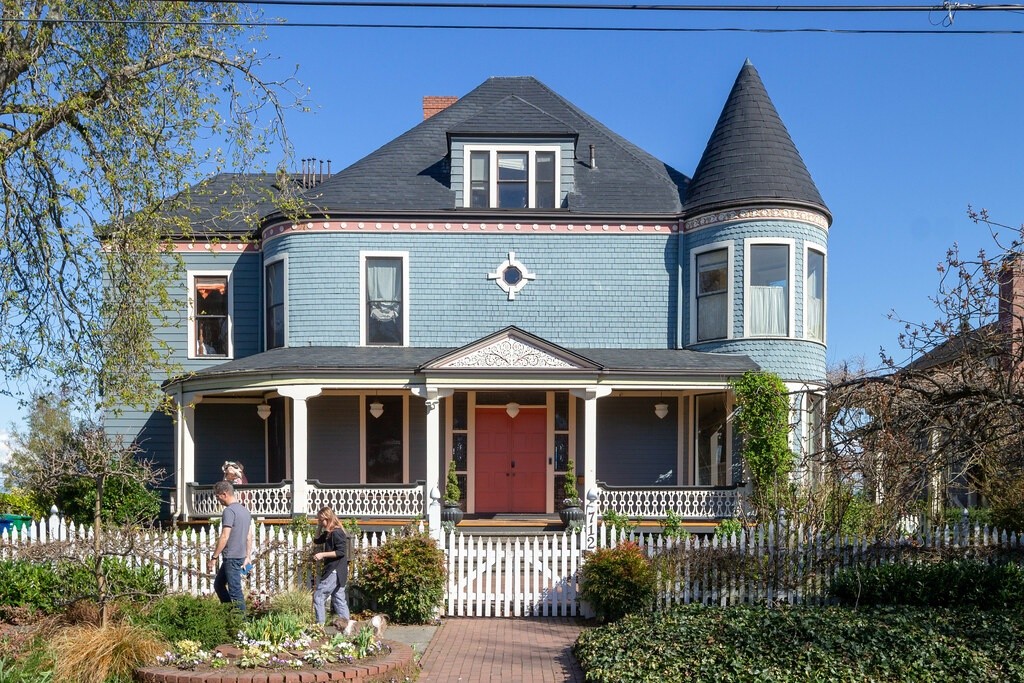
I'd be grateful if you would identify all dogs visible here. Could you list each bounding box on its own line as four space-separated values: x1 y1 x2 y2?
328 614 390 640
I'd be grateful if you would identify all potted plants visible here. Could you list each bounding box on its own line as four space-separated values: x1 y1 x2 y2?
558 458 584 526
441 460 463 527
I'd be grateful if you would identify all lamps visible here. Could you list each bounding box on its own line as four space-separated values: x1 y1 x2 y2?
506 402 520 419
654 391 668 419
257 394 271 420
370 391 384 418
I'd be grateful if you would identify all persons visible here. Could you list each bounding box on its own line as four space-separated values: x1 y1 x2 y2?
312 507 350 627
206 482 252 621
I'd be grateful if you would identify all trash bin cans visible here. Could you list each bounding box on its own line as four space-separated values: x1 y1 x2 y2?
0 514 33 536
0 519 14 536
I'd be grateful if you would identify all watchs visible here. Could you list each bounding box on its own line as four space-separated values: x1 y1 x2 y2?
211 555 217 560
